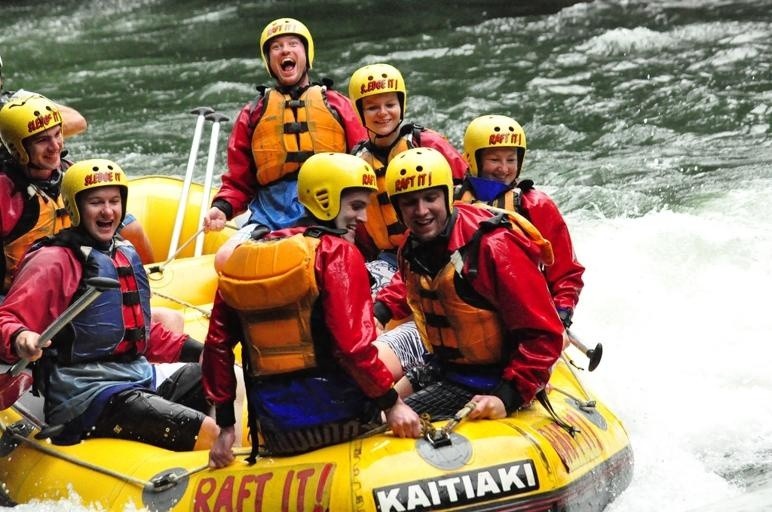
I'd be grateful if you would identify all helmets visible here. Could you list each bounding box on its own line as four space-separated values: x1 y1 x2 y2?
296 151 380 222
0 92 64 166
464 113 528 180
259 17 316 79
60 158 129 227
349 63 407 128
385 146 455 227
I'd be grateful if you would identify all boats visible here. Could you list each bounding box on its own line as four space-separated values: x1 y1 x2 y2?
0 175 633 512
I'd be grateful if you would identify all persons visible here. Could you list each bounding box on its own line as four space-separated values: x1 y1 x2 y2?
1 88 247 452
201 17 587 471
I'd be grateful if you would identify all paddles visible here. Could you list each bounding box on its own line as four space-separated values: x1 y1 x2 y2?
0 279 117 411
